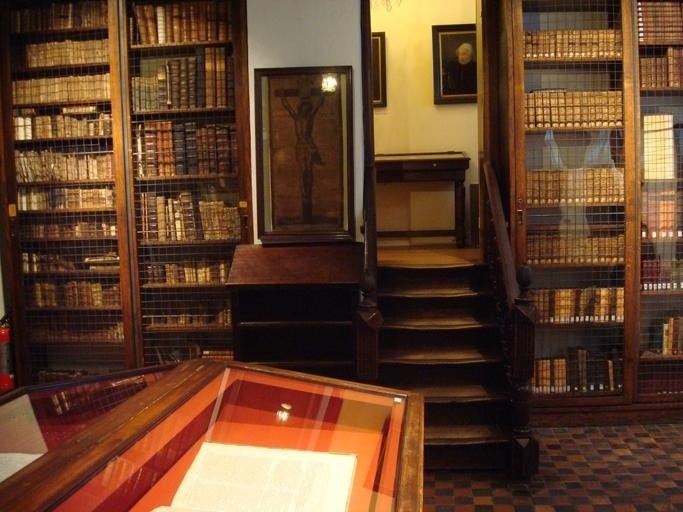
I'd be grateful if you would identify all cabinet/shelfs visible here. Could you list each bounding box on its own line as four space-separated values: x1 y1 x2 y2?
491 0 682 413
226 241 365 387
9 2 250 389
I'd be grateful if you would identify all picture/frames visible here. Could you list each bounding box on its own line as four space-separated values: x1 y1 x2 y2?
371 31 388 112
252 64 353 242
431 23 477 103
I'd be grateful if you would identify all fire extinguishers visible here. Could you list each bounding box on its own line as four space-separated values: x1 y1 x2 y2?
0 314 16 394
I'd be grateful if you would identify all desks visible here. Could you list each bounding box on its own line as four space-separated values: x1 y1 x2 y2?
373 147 470 248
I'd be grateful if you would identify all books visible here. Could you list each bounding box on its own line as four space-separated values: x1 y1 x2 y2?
523 28 624 394
636 1 683 401
7 1 126 381
128 2 242 359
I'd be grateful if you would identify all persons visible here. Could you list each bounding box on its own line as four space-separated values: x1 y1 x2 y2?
447 42 476 93
277 87 325 217
